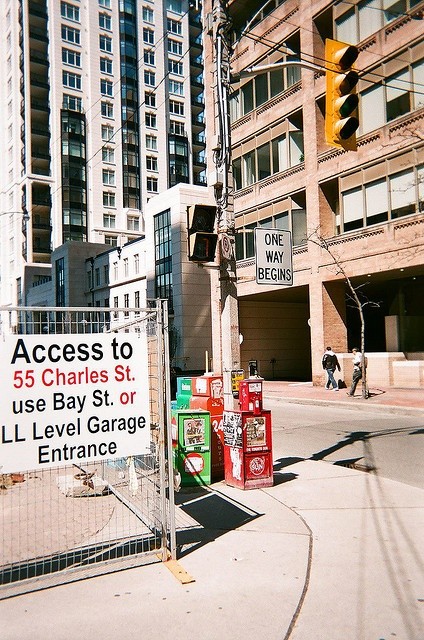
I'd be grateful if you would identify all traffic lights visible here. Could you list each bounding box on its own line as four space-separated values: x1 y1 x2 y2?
186 205 216 262
323 38 360 154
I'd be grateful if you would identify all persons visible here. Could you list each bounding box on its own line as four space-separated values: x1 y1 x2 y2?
346 347 362 397
322 347 341 391
194 423 202 443
187 423 195 444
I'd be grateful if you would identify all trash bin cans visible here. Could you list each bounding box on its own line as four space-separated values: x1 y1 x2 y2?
248 360 260 376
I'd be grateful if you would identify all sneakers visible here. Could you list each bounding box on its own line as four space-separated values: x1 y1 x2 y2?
346 392 354 397
324 385 330 390
332 387 339 391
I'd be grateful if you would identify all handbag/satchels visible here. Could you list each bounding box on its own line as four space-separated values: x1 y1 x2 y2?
360 356 368 367
